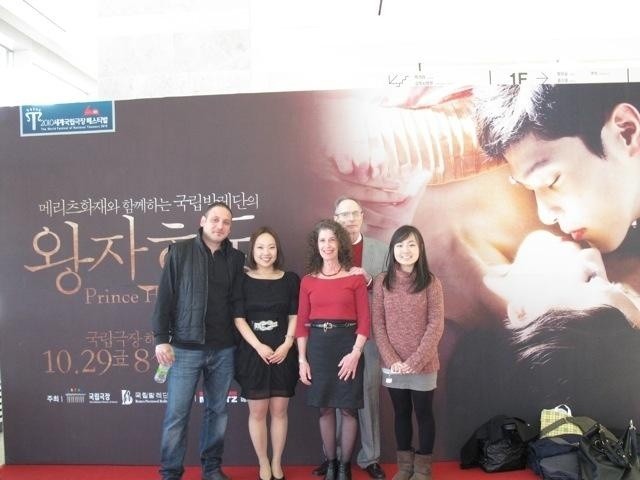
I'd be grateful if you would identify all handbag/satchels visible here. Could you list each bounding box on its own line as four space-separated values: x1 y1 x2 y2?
460 408 640 480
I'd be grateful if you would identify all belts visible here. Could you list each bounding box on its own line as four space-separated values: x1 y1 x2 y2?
308 321 358 333
246 318 288 332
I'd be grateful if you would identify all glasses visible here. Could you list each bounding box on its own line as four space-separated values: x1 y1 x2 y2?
335 210 362 218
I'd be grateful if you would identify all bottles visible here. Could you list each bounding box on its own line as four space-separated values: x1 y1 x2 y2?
153 359 172 384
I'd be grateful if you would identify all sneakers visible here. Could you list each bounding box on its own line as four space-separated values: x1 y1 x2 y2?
201 467 230 480
163 473 181 480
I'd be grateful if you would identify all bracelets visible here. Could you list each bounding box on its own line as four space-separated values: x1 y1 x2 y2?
283 334 296 341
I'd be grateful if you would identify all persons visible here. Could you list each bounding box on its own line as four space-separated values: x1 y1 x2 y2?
311 82 640 438
295 218 371 479
370 224 445 480
153 205 248 479
229 225 299 479
311 195 391 479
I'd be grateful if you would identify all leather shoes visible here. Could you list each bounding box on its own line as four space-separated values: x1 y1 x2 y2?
366 462 387 480
336 459 353 480
258 459 285 480
312 458 340 476
323 458 338 480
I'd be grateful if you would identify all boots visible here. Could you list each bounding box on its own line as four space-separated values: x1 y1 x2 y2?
391 448 415 480
409 450 434 480
